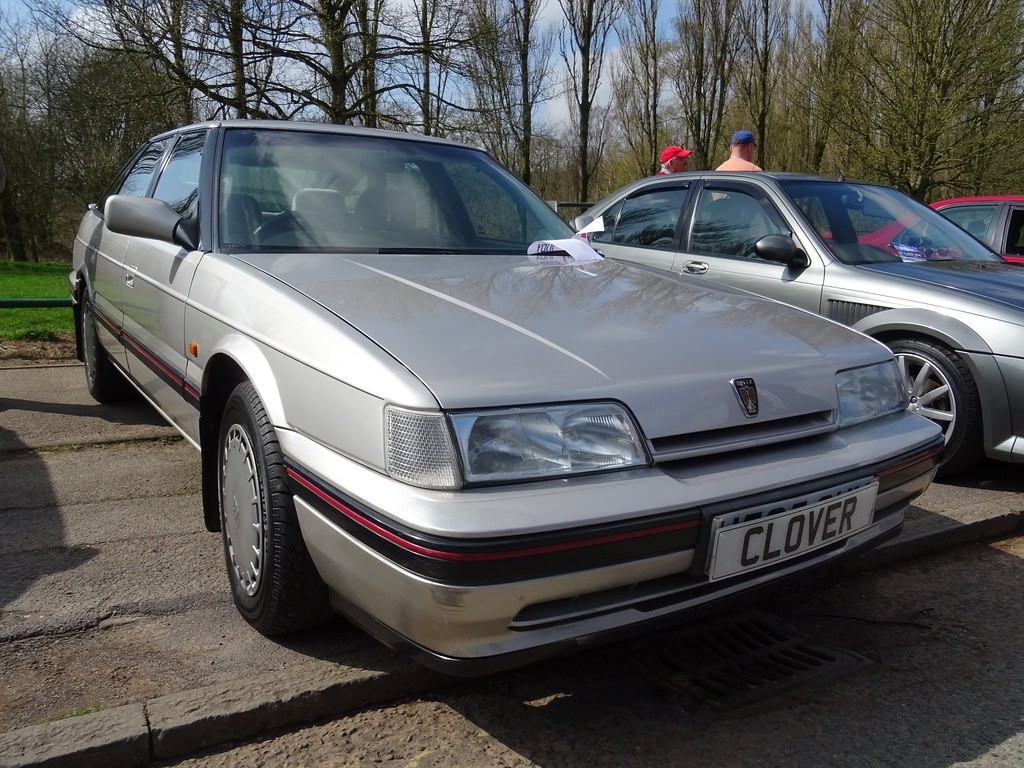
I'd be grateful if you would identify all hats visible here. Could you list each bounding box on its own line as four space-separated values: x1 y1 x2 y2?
730 130 757 147
660 146 692 164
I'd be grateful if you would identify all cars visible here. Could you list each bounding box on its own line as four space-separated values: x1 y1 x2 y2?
822 195 1024 264
535 172 1024 469
67 119 945 681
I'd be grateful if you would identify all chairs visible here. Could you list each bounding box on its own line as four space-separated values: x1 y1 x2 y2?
227 186 440 247
702 197 743 254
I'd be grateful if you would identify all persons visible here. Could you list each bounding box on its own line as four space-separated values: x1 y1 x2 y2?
656 145 692 175
715 129 762 172
